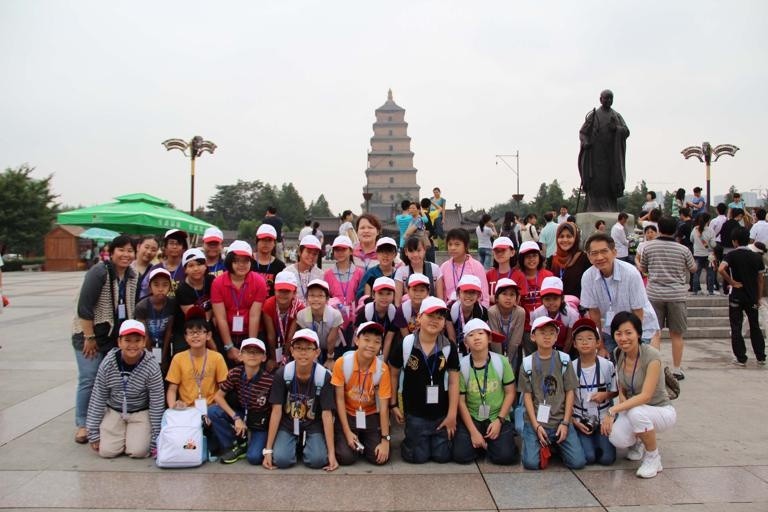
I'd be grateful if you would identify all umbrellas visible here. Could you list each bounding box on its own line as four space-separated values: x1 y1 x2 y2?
53 191 219 240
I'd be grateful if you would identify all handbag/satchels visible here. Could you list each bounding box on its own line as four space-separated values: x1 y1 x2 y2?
664 367 680 400
712 254 719 273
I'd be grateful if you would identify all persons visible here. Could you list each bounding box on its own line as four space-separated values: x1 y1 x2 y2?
72 186 676 479
640 185 768 380
578 88 631 213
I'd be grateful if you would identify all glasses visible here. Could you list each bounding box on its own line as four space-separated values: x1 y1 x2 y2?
588 248 612 257
186 330 208 336
293 345 317 352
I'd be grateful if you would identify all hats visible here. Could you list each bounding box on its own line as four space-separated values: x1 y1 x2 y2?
274 271 298 292
149 268 170 282
376 237 397 251
407 237 601 343
181 249 207 266
119 319 146 338
256 224 277 241
373 276 395 292
240 337 266 356
292 328 319 350
300 235 322 251
333 236 354 250
202 228 224 243
227 240 252 257
357 320 384 336
308 278 330 290
165 228 188 238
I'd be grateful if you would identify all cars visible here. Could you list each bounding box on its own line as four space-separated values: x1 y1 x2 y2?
3 254 22 263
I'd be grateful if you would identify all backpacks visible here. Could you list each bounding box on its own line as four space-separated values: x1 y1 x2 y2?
513 351 571 433
154 407 208 468
501 223 518 248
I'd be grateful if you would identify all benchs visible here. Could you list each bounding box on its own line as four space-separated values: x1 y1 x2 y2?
22 264 43 273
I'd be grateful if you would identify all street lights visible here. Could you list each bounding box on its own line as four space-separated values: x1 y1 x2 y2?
493 150 521 212
364 147 392 214
161 135 218 215
683 139 740 210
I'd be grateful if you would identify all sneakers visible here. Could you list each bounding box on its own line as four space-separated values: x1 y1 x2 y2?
626 441 644 461
732 358 746 367
758 359 766 365
220 443 248 465
672 370 684 380
74 428 89 444
636 454 664 478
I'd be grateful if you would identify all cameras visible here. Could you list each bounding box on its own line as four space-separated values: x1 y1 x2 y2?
580 414 598 431
202 299 212 312
